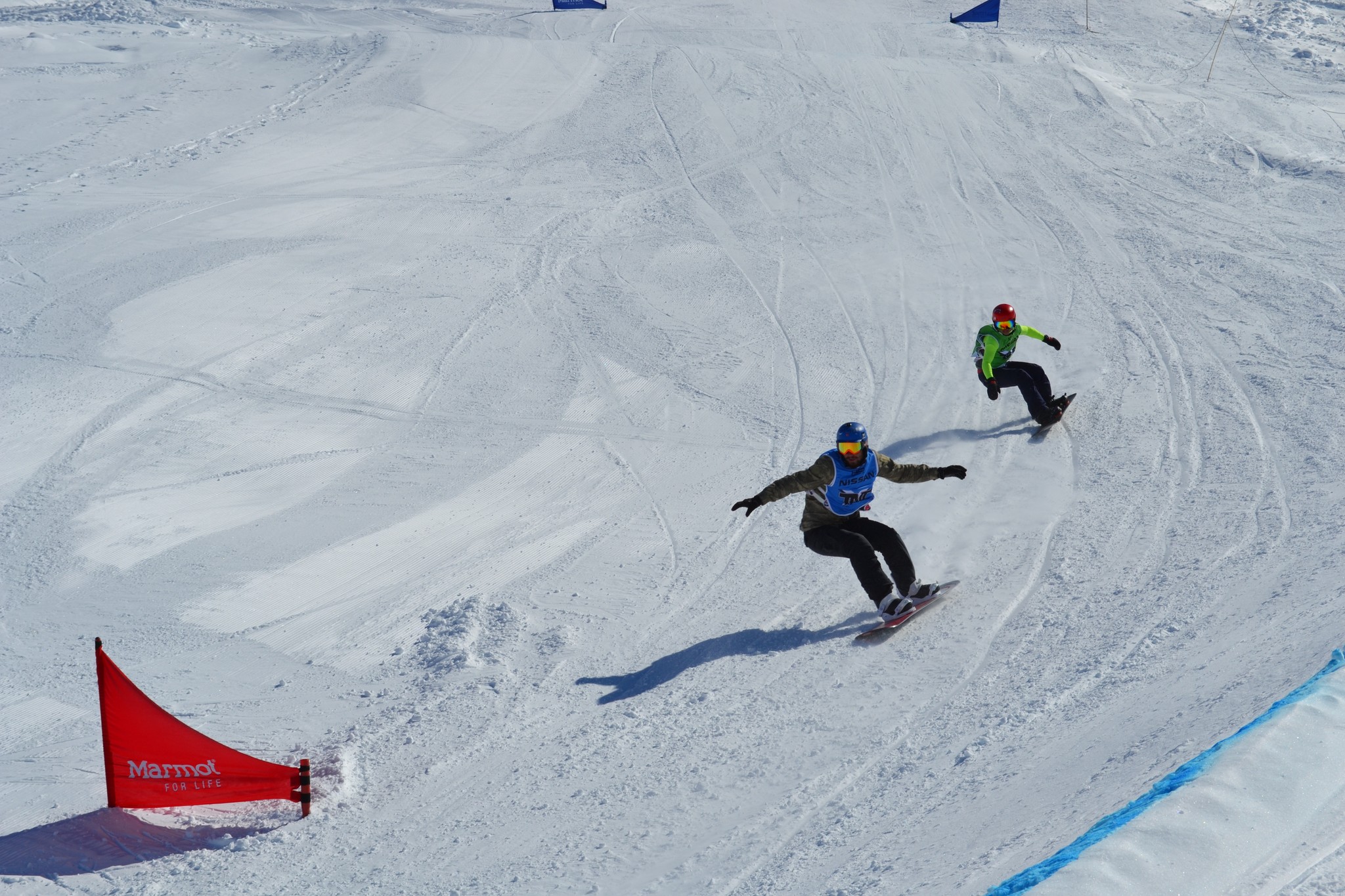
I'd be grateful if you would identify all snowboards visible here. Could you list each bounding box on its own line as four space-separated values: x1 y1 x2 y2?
1031 393 1077 437
856 580 960 638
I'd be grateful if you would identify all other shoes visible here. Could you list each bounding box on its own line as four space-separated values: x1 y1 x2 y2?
898 583 940 599
874 597 913 615
1036 405 1062 426
1048 395 1068 408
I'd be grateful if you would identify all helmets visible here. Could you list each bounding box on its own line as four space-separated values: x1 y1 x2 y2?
992 304 1016 325
836 422 868 443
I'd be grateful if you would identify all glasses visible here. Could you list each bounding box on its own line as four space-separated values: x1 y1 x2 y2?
837 440 865 455
994 318 1016 330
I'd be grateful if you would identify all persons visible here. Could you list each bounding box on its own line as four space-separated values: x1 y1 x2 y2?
971 303 1071 426
732 422 967 623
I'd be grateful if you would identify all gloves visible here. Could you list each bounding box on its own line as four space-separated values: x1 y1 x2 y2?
986 377 1001 400
938 465 967 480
1042 334 1061 350
731 495 761 517
